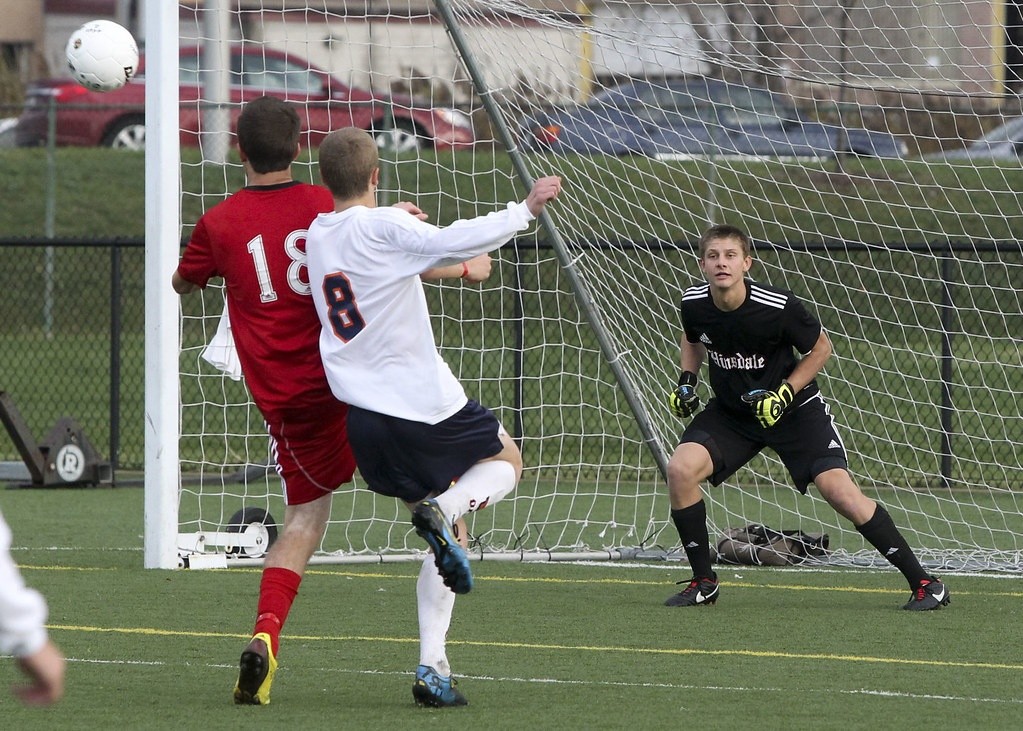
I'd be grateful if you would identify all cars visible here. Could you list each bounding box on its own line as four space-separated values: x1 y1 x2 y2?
916 115 1023 164
513 71 911 162
14 41 476 157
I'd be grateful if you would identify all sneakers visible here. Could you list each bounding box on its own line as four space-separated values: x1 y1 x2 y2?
231 633 278 706
664 570 720 606
409 498 474 595
901 575 951 612
412 663 470 707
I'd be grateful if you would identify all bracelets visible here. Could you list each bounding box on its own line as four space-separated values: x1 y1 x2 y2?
460 262 468 279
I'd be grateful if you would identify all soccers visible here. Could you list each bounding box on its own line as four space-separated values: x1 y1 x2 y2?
64 20 140 92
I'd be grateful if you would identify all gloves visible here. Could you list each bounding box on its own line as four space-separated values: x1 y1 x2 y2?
740 381 795 430
669 385 699 419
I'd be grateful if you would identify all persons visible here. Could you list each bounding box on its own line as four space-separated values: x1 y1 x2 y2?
305 127 563 710
169 97 491 701
664 225 951 611
0 510 70 706
